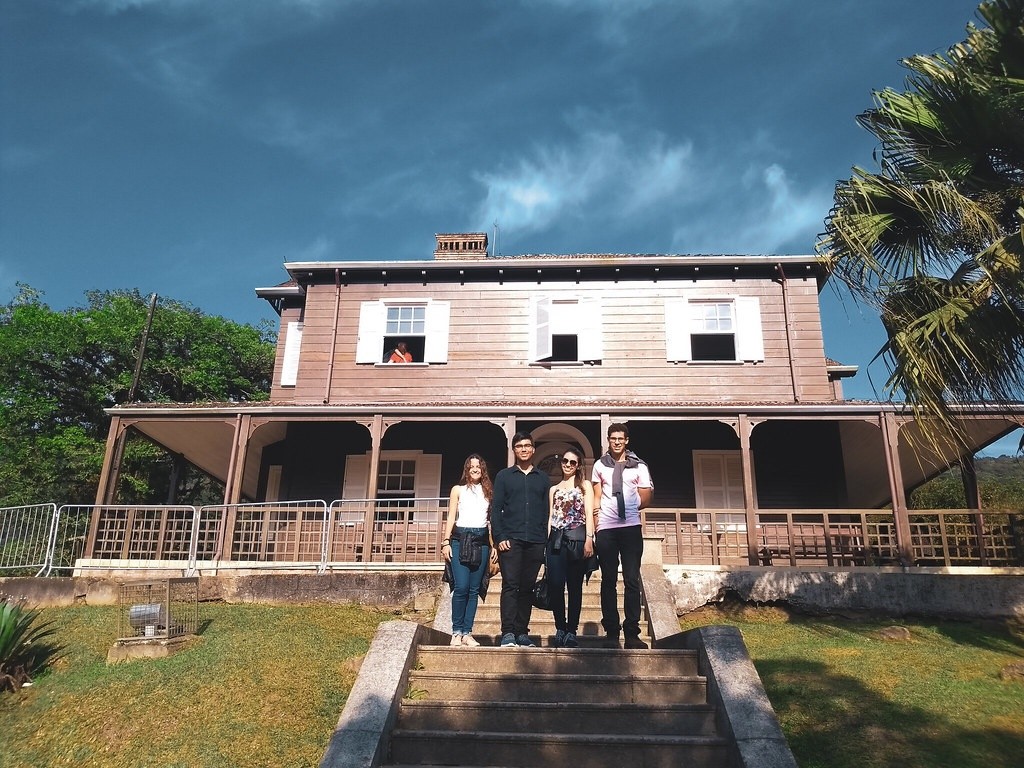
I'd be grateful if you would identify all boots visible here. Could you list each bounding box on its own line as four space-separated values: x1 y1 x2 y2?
624 630 648 649
604 629 620 648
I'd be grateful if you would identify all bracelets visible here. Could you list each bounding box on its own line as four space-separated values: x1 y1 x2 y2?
585 533 594 538
441 538 451 549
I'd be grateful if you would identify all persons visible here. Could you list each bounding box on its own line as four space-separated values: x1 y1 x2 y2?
490 430 553 647
591 423 654 649
911 517 937 556
384 341 411 363
440 453 498 647
872 520 896 555
546 447 594 648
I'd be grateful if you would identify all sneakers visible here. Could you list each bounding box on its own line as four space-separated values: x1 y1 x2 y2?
563 630 579 648
553 630 565 647
462 632 480 646
501 633 517 646
450 633 463 646
516 634 536 646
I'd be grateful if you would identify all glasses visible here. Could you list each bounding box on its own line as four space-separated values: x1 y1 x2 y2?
561 458 578 467
514 444 533 451
608 437 627 444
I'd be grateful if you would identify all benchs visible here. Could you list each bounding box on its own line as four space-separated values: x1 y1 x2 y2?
759 548 900 566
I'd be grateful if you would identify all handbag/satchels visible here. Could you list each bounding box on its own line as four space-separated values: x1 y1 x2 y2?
486 534 499 577
529 555 553 610
